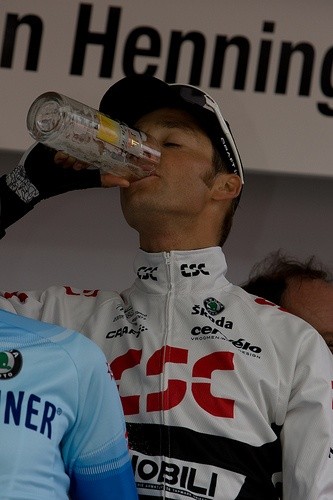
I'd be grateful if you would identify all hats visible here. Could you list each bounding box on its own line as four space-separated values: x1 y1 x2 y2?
99 73 244 205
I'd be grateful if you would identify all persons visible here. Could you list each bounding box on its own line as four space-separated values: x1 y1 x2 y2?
0 309 139 500
0 75 333 500
239 247 333 356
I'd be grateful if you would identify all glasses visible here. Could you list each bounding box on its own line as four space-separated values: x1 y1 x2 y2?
168 80 245 185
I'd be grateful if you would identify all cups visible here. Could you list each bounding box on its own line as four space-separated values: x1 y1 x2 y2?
26 91 162 182
